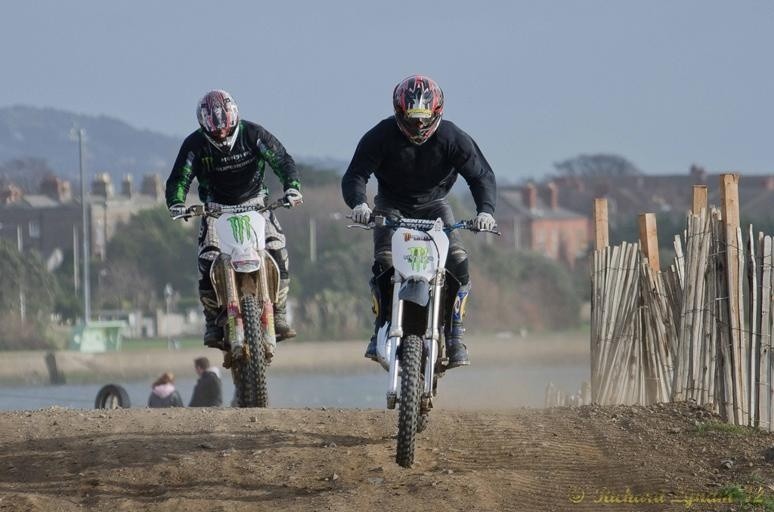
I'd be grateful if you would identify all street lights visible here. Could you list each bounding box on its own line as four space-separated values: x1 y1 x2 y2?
67 126 91 326
162 281 170 350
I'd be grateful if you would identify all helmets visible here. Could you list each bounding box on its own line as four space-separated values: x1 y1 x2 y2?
393 75 445 146
195 88 240 154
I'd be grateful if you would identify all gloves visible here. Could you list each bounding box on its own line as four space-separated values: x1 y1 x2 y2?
352 203 374 226
283 188 304 208
169 204 188 222
473 211 496 232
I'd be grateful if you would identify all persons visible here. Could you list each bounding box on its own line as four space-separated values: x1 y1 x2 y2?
188 357 224 407
165 89 302 348
148 370 184 408
339 77 498 368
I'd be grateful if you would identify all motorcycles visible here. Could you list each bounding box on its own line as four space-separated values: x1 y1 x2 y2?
170 194 303 409
345 212 502 468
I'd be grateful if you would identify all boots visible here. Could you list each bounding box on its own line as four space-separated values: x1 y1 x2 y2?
199 289 224 345
365 277 392 361
442 279 472 366
273 277 291 333
